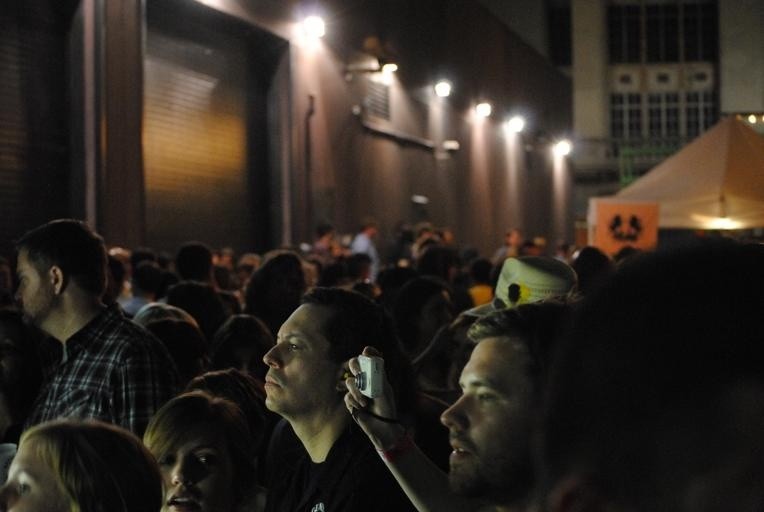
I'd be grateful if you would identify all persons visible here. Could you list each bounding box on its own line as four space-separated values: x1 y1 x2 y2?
0 215 639 512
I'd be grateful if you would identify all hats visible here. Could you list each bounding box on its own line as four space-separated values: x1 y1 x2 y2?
459 255 586 323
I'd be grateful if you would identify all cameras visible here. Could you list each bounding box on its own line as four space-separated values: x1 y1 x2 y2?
355 352 384 400
